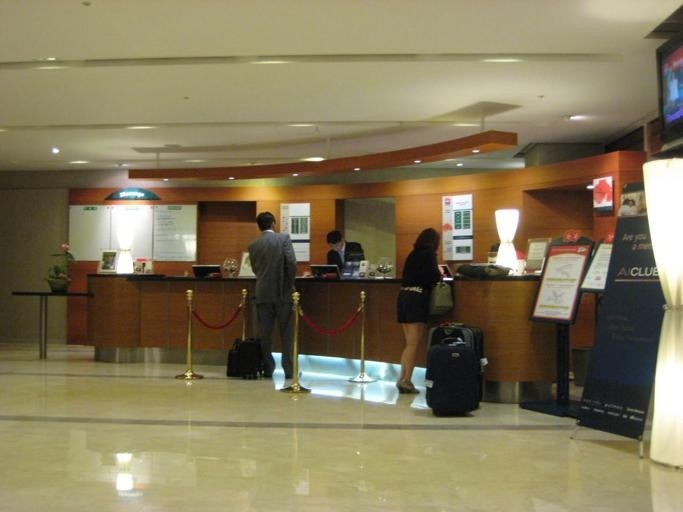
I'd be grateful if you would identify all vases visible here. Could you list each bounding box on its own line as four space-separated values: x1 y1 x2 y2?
45 276 68 292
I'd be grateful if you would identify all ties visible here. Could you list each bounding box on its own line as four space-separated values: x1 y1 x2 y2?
340 252 345 265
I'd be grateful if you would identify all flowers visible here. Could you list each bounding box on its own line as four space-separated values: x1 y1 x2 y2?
49 242 78 283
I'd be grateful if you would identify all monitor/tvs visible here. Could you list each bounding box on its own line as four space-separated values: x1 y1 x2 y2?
310 264 341 279
192 265 221 277
655 31 683 142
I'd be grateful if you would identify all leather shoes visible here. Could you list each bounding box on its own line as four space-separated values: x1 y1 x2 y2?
284 382 311 393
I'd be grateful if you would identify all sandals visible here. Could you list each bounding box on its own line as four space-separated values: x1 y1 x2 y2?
396 379 419 394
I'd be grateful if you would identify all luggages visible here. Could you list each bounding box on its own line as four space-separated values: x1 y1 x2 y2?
426 322 488 417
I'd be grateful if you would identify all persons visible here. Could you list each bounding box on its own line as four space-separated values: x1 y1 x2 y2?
249 212 301 378
327 230 364 273
397 228 441 394
618 198 638 217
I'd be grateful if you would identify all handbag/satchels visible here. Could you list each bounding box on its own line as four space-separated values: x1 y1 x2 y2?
428 276 453 316
227 338 276 379
